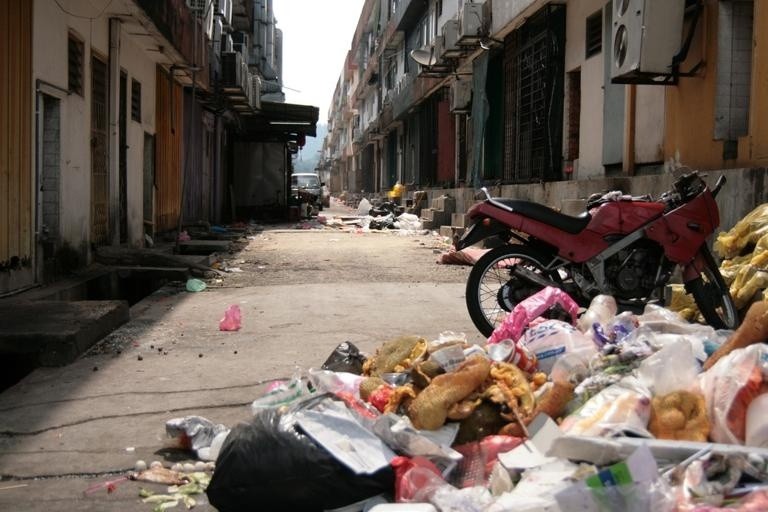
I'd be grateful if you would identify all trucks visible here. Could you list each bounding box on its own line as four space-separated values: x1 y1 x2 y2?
290 173 324 210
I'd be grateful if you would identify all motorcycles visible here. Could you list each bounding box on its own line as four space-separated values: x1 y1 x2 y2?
457 171 741 339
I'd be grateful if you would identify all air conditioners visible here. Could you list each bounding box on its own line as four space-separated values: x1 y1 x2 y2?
430 35 461 64
215 0 232 26
442 19 471 52
187 0 214 38
459 1 490 39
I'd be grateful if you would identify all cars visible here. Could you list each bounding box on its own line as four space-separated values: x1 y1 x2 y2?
321 185 331 209
